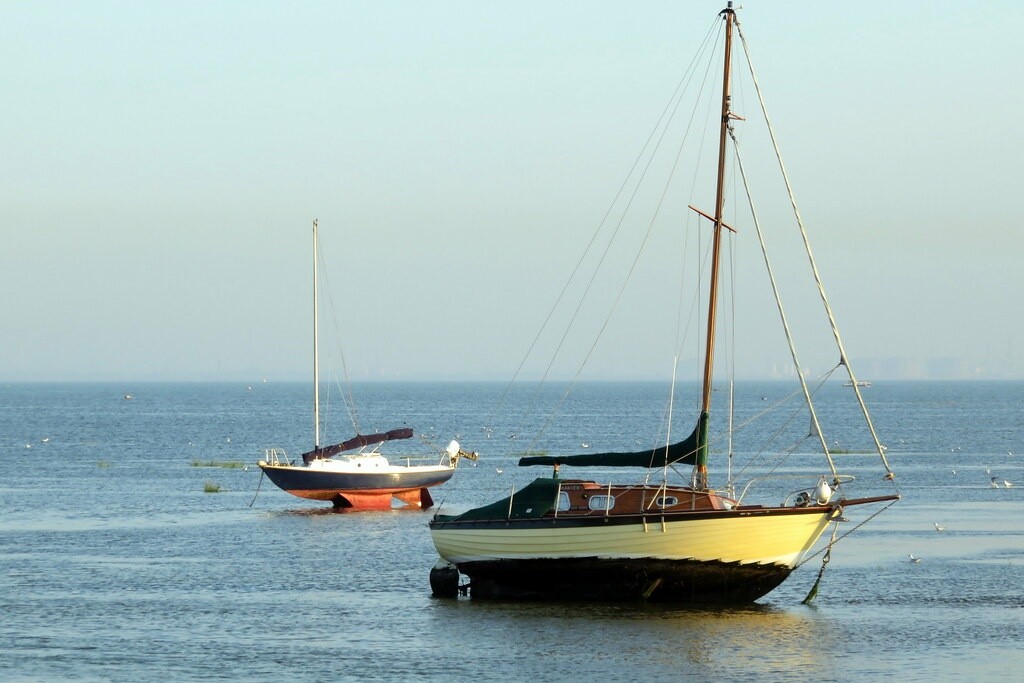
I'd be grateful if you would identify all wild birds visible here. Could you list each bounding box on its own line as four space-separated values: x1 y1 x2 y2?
0 379 1023 562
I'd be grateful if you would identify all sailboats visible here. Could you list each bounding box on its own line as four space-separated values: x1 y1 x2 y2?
431 1 902 607
254 218 464 514
